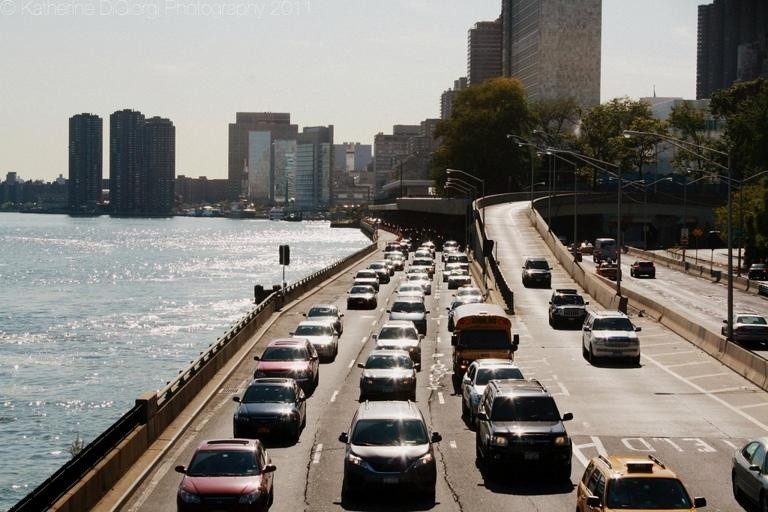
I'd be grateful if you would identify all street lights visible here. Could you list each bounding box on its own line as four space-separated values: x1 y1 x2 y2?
608 177 673 251
506 129 646 297
661 175 713 245
392 156 402 197
622 130 733 341
686 168 768 277
444 168 485 275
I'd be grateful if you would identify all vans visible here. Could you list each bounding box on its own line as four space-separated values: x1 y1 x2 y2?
582 309 641 364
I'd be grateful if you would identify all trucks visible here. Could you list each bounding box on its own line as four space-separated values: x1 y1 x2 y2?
451 303 519 387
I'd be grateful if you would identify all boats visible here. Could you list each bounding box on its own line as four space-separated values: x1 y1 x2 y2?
177 198 303 222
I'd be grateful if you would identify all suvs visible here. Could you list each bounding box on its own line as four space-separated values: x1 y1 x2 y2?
476 378 573 477
576 453 706 511
338 399 442 498
254 337 319 391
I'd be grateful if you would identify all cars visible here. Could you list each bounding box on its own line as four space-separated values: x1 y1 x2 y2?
232 377 306 443
748 263 767 280
630 261 656 280
372 319 425 368
461 358 525 430
732 436 768 512
289 320 339 362
174 438 276 511
357 350 421 399
721 312 768 348
